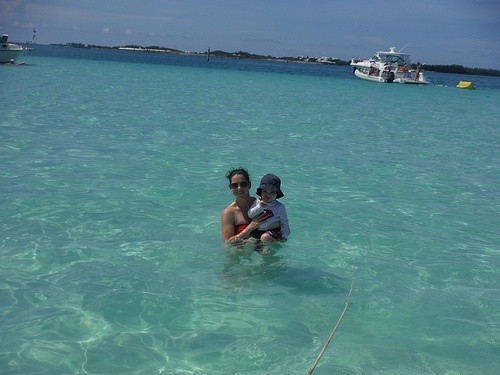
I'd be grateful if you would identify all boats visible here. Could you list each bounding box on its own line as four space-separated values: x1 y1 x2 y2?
350 44 428 85
0 29 37 67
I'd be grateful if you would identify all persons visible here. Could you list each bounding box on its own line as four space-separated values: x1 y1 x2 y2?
247 174 289 253
222 168 290 259
414 65 421 81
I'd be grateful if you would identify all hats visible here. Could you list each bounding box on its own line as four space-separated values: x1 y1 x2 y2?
256 174 284 198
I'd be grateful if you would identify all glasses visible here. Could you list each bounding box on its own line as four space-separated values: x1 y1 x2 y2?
231 182 249 188
